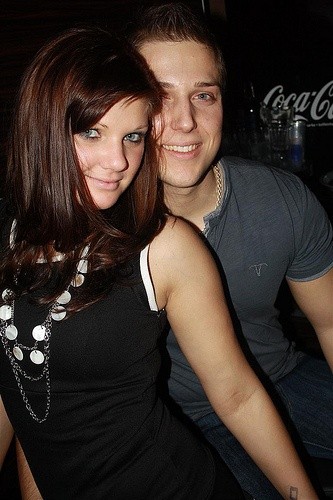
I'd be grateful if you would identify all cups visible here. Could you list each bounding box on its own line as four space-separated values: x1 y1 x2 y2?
286 121 304 162
262 106 294 162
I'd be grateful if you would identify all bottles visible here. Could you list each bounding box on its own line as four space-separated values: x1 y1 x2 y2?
242 81 265 160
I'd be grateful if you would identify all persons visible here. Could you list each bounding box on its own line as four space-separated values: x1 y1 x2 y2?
15 10 333 500
0 29 319 500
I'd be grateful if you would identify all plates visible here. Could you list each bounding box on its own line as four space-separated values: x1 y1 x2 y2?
320 172 333 190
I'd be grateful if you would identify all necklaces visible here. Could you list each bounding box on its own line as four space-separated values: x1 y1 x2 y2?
213 162 223 209
0 243 92 423
